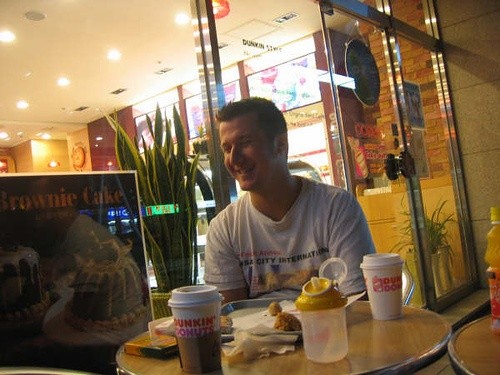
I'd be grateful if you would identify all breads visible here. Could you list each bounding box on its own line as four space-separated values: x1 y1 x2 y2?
274 312 302 331
220 316 233 333
269 301 282 315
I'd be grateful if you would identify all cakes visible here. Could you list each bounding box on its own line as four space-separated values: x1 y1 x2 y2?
0 241 49 321
64 229 151 333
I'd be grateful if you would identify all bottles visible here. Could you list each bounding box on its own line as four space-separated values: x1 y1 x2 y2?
294 257 349 363
484 206 500 335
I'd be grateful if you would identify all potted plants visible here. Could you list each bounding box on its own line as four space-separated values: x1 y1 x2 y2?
389 193 459 297
103 106 215 319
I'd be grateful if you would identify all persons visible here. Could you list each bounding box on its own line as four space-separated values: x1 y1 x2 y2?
28 180 146 304
204 97 376 301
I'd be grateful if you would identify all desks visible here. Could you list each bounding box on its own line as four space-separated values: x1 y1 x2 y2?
114 301 451 375
447 314 500 375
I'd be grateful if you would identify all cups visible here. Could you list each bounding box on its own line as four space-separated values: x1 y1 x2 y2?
359 253 404 320
167 285 224 374
